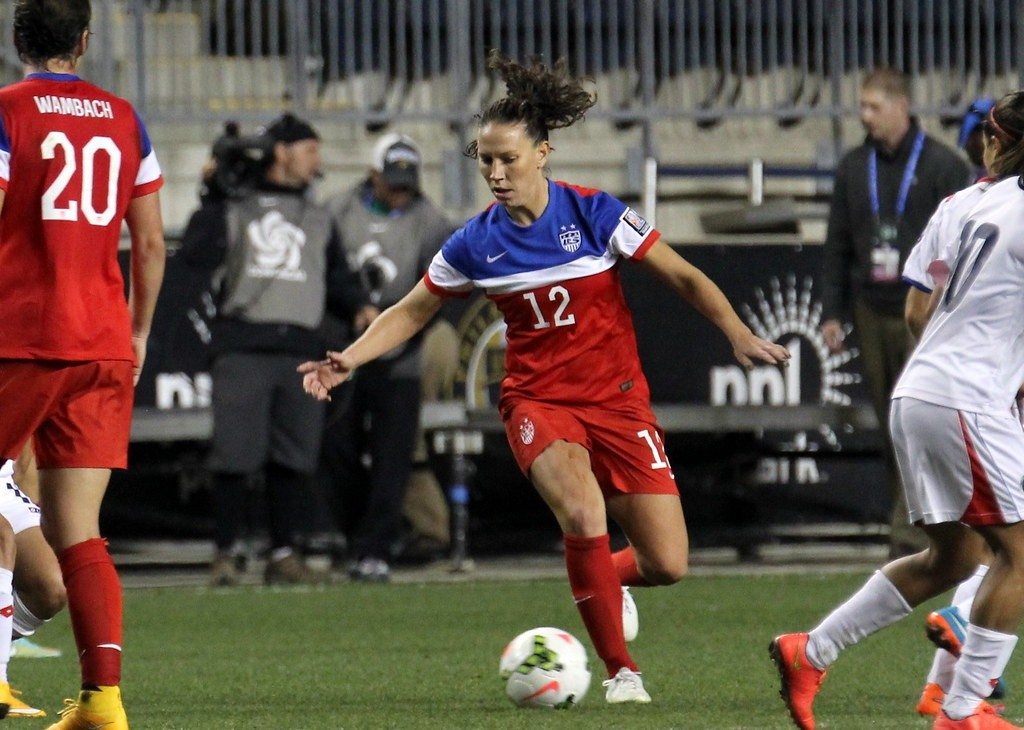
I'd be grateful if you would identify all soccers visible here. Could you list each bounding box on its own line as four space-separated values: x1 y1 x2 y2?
496 626 594 711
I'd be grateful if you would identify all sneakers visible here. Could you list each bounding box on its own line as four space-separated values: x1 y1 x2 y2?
0 680 47 716
768 633 826 730
44 697 129 730
602 666 651 703
933 698 1024 730
927 605 1006 699
621 586 638 642
917 682 946 715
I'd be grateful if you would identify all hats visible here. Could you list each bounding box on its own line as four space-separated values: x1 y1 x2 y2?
262 118 319 145
372 134 421 190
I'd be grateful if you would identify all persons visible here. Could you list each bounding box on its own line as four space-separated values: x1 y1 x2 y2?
298 51 794 703
309 130 487 584
818 68 974 566
904 91 1010 716
179 110 379 582
765 170 1021 730
0 2 172 730
0 425 69 718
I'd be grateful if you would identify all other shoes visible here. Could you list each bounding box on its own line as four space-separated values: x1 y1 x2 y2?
207 554 239 587
265 553 342 587
352 556 390 581
